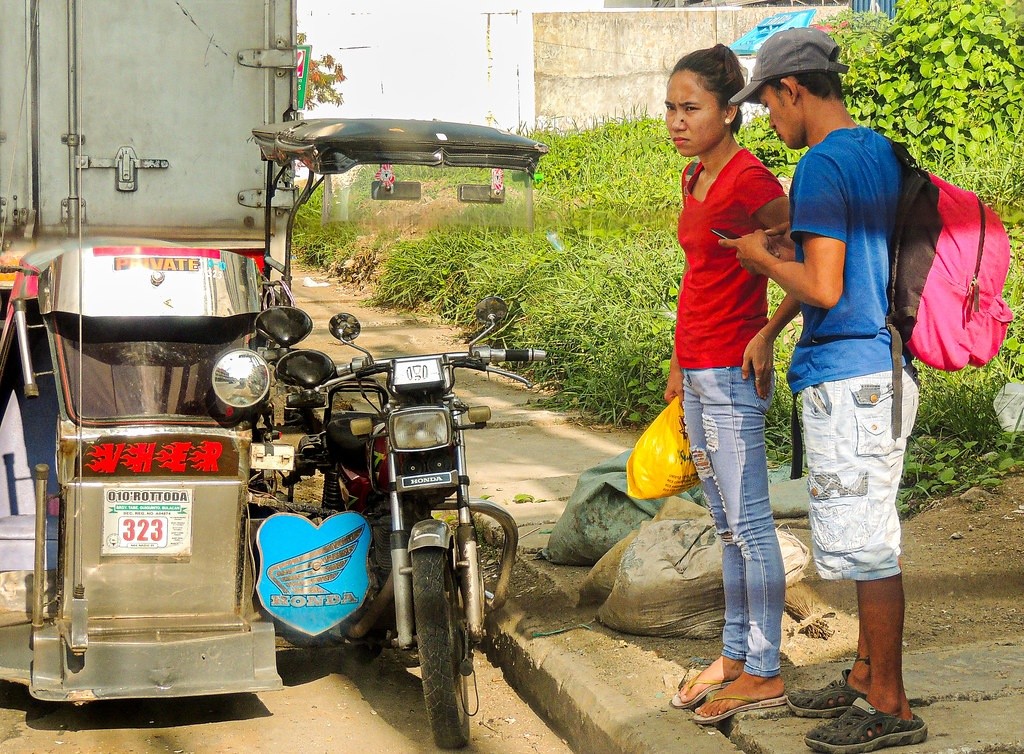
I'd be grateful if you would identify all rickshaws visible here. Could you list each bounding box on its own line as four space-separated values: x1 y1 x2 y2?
0 119 548 751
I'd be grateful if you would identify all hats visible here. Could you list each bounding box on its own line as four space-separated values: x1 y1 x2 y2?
728 25 848 107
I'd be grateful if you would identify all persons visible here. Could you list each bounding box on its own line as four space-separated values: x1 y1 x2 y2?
664 44 795 724
717 25 928 754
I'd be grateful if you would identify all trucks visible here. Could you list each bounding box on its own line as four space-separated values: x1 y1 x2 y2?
0 1 299 291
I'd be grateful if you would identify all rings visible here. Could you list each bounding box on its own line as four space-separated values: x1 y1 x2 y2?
755 377 761 381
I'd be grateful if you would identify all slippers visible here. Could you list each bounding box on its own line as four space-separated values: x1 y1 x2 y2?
693 688 789 725
805 698 930 754
671 671 735 709
784 667 870 718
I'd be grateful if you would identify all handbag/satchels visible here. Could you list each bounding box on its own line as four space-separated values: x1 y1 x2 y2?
625 393 701 501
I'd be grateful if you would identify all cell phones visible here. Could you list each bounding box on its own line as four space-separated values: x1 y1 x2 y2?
709 227 742 240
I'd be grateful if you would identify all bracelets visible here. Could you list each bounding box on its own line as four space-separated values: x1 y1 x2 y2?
758 332 774 345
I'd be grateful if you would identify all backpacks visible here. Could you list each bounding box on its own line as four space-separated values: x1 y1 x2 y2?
883 135 1015 372
